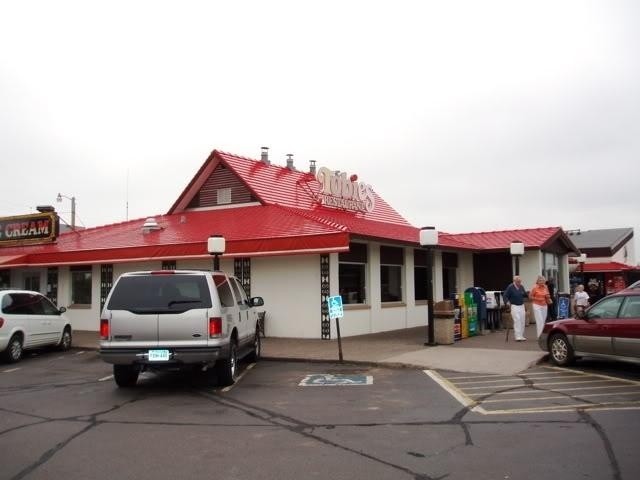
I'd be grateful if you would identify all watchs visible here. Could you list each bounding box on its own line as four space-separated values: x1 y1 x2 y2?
528 275 554 338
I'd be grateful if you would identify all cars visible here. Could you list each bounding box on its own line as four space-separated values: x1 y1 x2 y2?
595 281 640 309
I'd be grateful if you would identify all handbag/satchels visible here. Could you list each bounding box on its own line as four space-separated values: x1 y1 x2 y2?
546 298 553 303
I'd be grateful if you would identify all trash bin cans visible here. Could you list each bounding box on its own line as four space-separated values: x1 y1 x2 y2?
432 300 456 345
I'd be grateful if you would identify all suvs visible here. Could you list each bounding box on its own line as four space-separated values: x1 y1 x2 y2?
535 290 640 369
98 268 268 390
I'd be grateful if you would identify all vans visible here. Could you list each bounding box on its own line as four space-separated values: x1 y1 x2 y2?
0 287 74 361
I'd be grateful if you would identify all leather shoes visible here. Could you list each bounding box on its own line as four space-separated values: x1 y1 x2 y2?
515 337 526 341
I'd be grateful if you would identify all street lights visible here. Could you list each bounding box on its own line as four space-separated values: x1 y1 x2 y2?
205 234 226 270
576 252 588 290
55 192 78 233
418 225 442 344
510 241 526 278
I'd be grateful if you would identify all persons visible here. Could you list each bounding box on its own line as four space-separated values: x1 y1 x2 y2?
503 275 530 340
574 284 590 312
